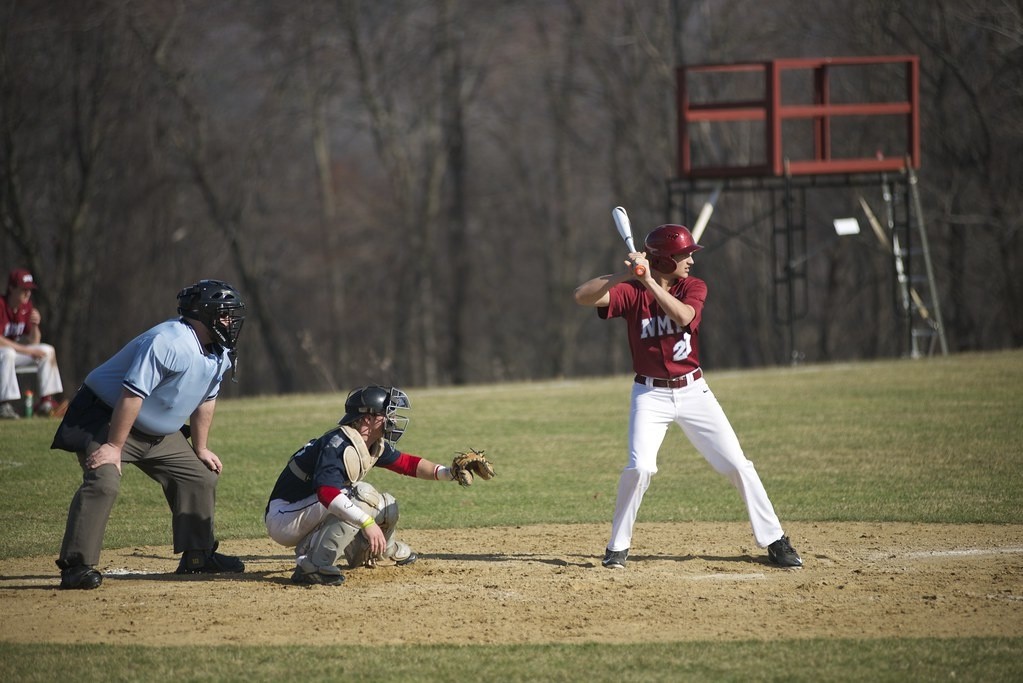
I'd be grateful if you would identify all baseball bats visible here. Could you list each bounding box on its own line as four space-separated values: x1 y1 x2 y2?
611 206 646 277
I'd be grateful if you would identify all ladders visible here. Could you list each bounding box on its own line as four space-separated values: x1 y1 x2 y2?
877 151 949 358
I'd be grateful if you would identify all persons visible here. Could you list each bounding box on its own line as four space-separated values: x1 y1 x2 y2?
51 279 247 589
0 269 63 417
263 384 496 586
574 224 803 567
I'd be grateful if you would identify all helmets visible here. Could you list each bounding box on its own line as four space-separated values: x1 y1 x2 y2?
643 224 704 273
338 386 390 425
176 279 245 383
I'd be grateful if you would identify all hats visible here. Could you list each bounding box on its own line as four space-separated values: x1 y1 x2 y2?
9 268 38 289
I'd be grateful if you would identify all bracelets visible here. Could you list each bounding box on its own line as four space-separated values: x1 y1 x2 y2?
361 518 374 529
434 465 455 481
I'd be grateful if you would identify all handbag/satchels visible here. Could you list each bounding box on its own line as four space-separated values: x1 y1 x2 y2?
50 395 107 452
180 424 192 440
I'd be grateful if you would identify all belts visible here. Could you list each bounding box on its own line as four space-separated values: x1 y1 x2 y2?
79 384 162 439
635 369 701 388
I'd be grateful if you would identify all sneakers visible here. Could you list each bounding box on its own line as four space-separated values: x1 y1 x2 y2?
768 535 803 569
54 559 102 590
174 539 244 574
394 552 416 565
602 547 628 569
291 565 346 586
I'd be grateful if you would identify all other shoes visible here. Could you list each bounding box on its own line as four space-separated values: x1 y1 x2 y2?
39 400 55 418
0 403 19 420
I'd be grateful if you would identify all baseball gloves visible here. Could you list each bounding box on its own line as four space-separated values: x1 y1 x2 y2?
452 452 495 487
